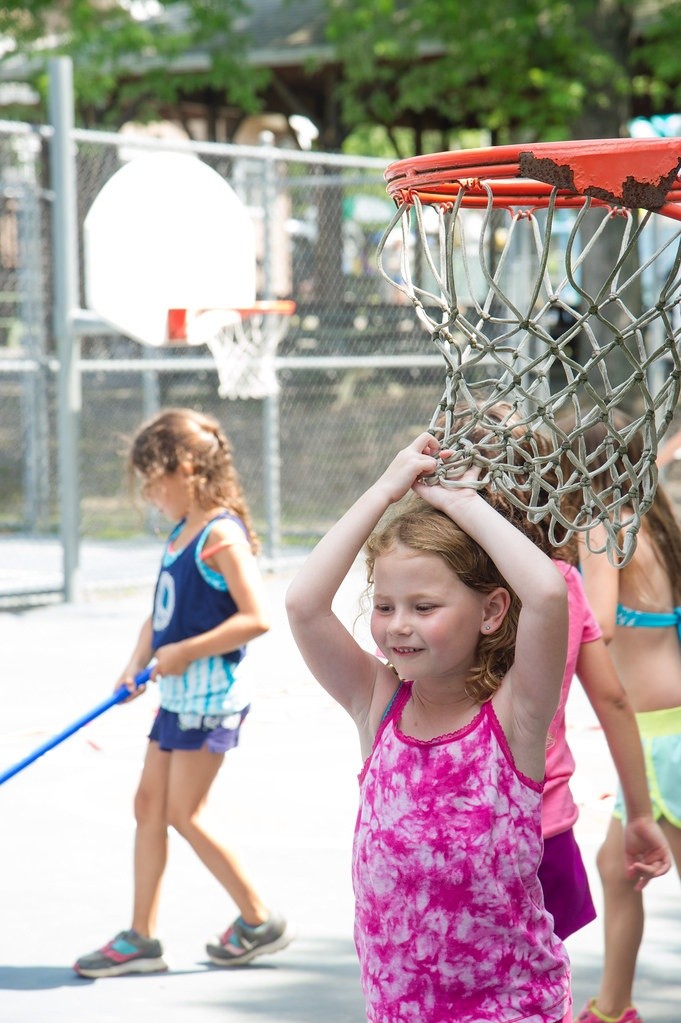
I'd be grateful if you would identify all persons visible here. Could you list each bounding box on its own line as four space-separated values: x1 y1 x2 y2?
283 396 681 1023
72 408 291 978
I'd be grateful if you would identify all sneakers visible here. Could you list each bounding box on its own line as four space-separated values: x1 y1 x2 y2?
571 999 642 1023
207 912 290 967
73 931 168 978
